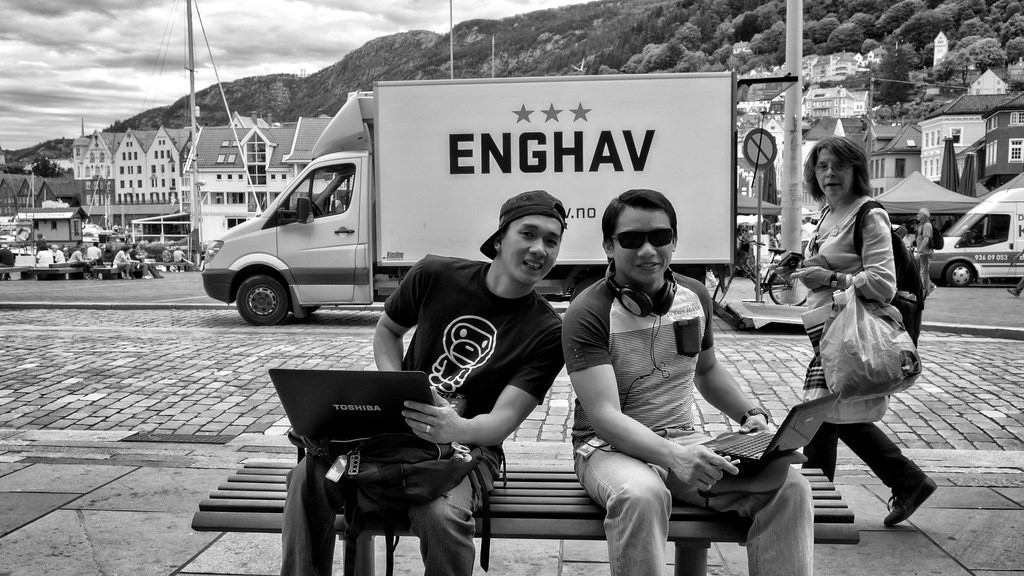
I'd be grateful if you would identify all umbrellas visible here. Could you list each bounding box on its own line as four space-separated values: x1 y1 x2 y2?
939 137 960 193
957 153 976 198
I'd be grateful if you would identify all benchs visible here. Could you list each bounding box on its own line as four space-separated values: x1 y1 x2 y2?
191 463 860 576
0 262 189 280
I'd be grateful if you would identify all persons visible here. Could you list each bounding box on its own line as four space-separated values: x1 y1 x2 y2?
802 217 818 268
36 234 102 280
281 190 565 576
102 242 164 280
155 247 186 273
0 244 15 281
199 249 206 272
767 137 936 527
895 218 952 251
916 208 937 299
705 268 719 291
736 225 755 278
1007 249 1024 296
562 190 814 576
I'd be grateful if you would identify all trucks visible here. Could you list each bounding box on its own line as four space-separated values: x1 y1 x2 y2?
202 72 736 325
929 172 1024 287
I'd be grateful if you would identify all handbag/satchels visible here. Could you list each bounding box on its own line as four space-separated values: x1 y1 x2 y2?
132 270 143 279
298 434 484 533
800 280 921 424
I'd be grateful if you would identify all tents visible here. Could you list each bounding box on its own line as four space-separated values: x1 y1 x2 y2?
736 194 782 215
874 171 981 214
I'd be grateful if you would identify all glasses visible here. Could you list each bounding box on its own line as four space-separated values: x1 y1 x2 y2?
811 159 851 176
611 228 674 250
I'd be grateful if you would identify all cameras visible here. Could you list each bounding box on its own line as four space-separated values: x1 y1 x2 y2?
778 251 803 269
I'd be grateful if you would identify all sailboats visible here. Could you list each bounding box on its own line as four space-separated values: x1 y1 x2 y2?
78 0 261 262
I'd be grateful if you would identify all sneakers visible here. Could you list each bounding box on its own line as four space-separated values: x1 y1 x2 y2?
884 476 938 526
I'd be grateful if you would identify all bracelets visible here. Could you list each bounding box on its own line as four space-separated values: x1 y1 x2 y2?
741 407 768 427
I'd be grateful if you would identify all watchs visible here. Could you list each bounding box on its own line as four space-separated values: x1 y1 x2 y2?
830 273 838 289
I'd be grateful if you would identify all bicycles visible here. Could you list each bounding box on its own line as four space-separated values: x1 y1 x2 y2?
712 224 812 306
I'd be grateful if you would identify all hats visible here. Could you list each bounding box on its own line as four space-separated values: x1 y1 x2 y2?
480 190 567 261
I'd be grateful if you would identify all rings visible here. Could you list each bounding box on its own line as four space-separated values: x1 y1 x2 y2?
426 425 430 433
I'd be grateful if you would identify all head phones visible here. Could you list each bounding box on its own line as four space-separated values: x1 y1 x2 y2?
605 260 677 318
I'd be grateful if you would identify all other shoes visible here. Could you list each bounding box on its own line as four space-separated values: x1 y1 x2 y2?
926 286 936 298
153 275 164 278
1007 288 1020 296
83 272 95 280
122 276 132 280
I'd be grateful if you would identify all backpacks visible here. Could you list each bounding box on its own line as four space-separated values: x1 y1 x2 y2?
818 201 923 347
917 222 944 250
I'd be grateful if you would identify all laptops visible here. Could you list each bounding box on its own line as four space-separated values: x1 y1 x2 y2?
267 367 466 445
699 390 843 466
